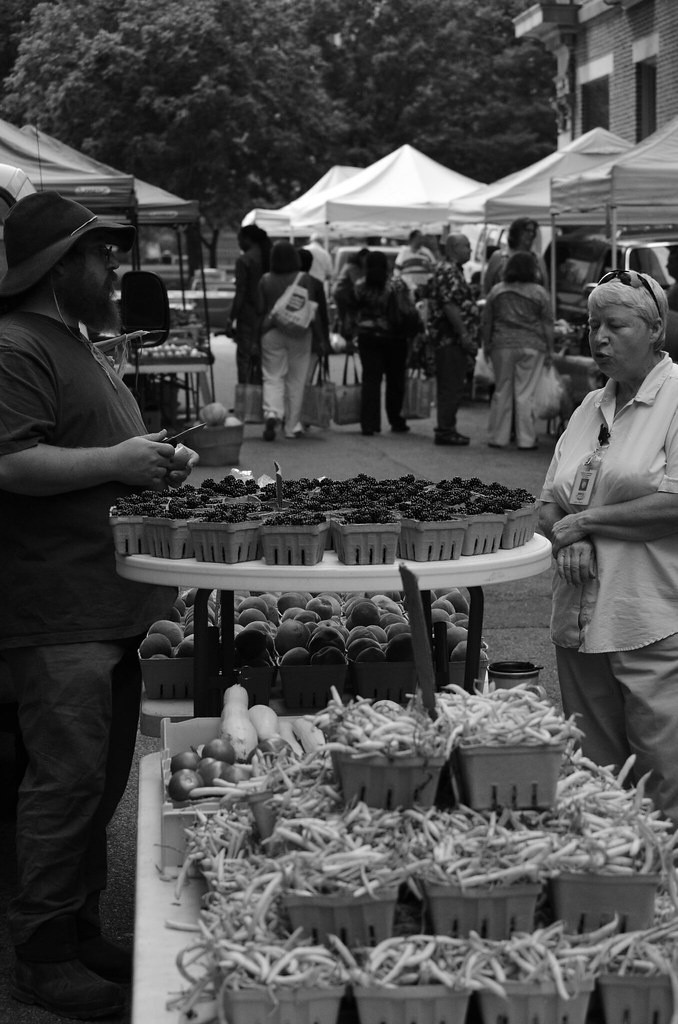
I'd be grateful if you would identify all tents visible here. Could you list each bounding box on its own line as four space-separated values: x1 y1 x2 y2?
0 119 139 269
19 125 215 401
450 126 635 286
289 144 487 294
242 165 366 245
548 114 678 318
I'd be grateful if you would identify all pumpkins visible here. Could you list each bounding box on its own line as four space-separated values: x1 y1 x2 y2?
199 403 227 424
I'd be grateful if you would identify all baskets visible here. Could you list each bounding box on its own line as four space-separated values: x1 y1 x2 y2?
215 735 672 1024
108 496 541 565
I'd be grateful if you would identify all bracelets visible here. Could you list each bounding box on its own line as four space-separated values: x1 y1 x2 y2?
461 333 468 340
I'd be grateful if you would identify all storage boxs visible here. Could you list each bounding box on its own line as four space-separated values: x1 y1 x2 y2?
110 504 546 564
136 597 676 1024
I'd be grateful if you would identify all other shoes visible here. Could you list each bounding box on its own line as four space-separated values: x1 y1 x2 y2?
362 427 381 436
434 432 470 446
391 425 410 433
82 942 135 982
8 950 134 1020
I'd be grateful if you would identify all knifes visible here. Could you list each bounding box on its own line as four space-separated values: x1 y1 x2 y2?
160 423 207 447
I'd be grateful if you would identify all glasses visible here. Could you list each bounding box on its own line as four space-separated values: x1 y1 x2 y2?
81 244 112 261
597 269 661 318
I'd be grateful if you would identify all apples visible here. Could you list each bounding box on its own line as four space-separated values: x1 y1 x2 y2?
139 344 208 357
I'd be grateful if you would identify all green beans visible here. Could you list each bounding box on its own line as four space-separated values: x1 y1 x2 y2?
168 748 678 1024
304 681 586 766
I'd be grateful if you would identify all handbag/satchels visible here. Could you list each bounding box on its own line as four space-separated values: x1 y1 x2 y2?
397 340 434 420
470 346 496 401
299 353 337 430
267 271 320 336
529 364 566 419
334 353 362 426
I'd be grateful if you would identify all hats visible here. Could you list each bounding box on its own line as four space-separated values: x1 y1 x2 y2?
0 190 137 303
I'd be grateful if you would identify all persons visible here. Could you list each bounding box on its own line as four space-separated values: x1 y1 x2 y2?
482 217 548 294
0 191 199 1023
480 251 555 449
224 224 438 441
426 232 480 446
538 271 678 834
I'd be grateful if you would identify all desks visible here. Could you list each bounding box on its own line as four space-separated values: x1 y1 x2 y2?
114 363 212 414
131 748 209 1024
114 529 558 715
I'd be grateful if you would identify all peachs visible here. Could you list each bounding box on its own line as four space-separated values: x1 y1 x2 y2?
140 588 487 666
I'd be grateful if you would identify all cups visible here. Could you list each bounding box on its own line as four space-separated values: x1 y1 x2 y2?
182 336 200 348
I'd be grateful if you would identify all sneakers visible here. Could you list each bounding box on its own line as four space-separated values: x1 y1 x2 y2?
285 428 303 440
262 411 277 442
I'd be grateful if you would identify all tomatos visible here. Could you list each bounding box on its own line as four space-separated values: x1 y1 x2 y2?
169 738 293 801
